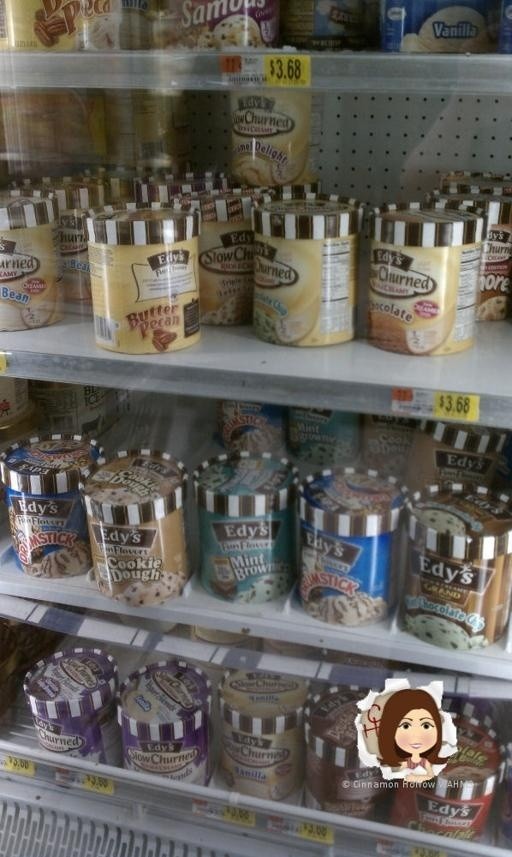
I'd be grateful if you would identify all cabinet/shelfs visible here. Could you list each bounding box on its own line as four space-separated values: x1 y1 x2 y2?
0 48 512 857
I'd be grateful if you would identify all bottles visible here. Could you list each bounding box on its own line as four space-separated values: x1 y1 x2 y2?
308 692 387 823
230 89 313 188
189 450 300 604
181 192 254 327
78 445 192 610
1 432 105 577
252 201 359 348
369 209 484 357
19 644 121 772
118 656 211 791
85 208 202 356
0 189 70 333
388 698 508 844
293 465 409 629
219 667 306 801
398 482 512 653
434 192 511 321
